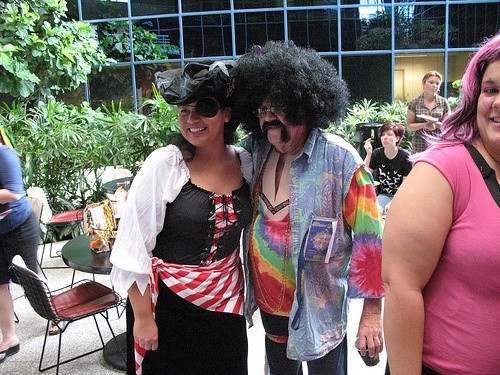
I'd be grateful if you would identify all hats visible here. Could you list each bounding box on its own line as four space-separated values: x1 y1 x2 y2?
154 60 237 105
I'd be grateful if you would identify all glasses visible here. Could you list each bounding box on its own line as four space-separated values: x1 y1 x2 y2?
253 106 280 117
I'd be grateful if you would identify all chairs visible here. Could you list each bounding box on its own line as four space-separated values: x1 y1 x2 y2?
26 187 84 266
11 255 127 375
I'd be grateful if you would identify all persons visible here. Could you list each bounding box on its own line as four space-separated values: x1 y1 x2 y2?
0 145 66 363
381 33 500 375
364 121 412 216
227 40 386 375
109 59 254 375
406 71 451 156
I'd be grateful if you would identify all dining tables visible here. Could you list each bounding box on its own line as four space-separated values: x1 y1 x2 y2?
61 236 127 319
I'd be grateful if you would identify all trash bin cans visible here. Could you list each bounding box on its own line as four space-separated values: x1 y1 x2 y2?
356 123 384 161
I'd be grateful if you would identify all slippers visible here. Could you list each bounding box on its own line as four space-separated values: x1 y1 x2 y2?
0 344 19 364
49 321 65 335
